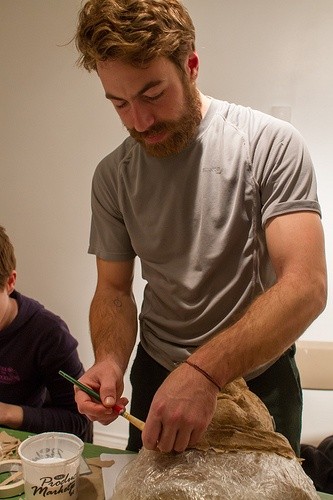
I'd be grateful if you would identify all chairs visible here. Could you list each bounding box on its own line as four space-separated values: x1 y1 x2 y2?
294 339 333 500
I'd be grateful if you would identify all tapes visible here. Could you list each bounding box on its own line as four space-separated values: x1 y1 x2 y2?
0 459 26 498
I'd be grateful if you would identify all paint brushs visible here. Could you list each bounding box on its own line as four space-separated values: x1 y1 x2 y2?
59 371 145 430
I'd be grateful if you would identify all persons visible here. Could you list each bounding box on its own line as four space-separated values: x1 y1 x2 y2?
73 0 327 454
0 226 95 446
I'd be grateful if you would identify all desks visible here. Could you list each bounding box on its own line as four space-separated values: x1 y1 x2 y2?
0 429 136 500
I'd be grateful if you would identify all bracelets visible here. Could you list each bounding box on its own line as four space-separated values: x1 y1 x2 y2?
182 358 224 393
